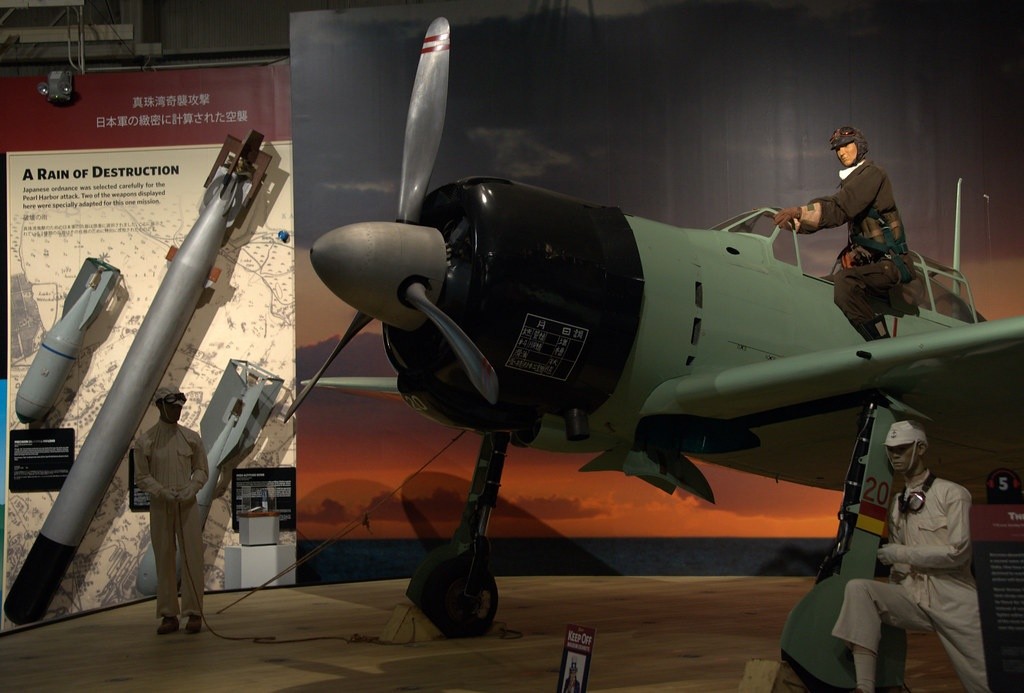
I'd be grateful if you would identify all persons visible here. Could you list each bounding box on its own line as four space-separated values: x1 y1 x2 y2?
773 127 910 342
134 383 209 636
830 420 989 693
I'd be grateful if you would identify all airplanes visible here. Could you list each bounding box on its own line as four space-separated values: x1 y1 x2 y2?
283 16 1024 693
